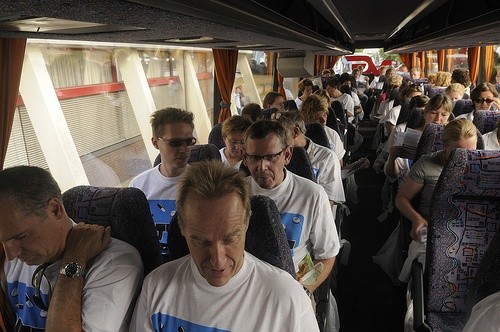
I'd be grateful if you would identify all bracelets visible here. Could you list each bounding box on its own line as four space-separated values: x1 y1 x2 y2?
304 287 311 299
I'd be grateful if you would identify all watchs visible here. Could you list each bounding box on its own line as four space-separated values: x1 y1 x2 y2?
59 261 85 278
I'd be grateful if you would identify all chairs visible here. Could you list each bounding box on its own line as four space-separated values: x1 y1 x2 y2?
207 122 224 149
164 194 291 275
152 144 224 170
284 99 298 111
381 68 500 332
363 73 375 84
262 108 277 118
331 99 346 121
62 187 164 274
303 122 330 149
290 146 318 184
326 106 340 133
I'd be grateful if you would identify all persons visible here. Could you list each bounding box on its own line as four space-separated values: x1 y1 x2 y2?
240 120 340 315
0 166 145 332
217 64 499 332
125 106 197 257
128 159 320 332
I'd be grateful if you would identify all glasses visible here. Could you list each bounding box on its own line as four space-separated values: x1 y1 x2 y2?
32 252 66 312
226 136 245 145
270 113 295 127
247 145 288 161
158 137 197 147
475 98 494 104
